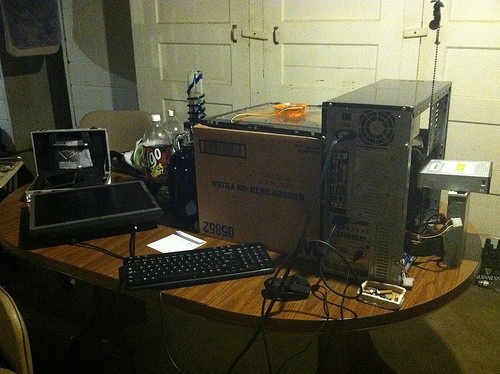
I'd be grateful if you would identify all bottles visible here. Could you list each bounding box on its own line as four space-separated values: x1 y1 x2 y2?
163 110 182 154
143 115 173 188
473 238 500 288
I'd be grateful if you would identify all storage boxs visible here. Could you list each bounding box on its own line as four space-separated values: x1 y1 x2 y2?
193 125 322 260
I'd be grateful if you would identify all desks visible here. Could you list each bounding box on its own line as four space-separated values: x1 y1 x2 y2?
0 170 482 374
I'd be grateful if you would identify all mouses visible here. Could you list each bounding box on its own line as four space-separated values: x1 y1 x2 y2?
262 272 312 300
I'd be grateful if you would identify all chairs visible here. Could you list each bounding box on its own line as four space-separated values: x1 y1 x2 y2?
0 286 33 374
80 110 152 154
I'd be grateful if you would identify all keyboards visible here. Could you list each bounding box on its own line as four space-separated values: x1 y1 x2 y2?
122 241 276 291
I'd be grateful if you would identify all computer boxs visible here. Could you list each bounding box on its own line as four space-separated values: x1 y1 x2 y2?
318 78 453 289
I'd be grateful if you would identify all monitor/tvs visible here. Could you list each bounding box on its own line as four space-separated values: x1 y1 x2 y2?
198 101 322 138
